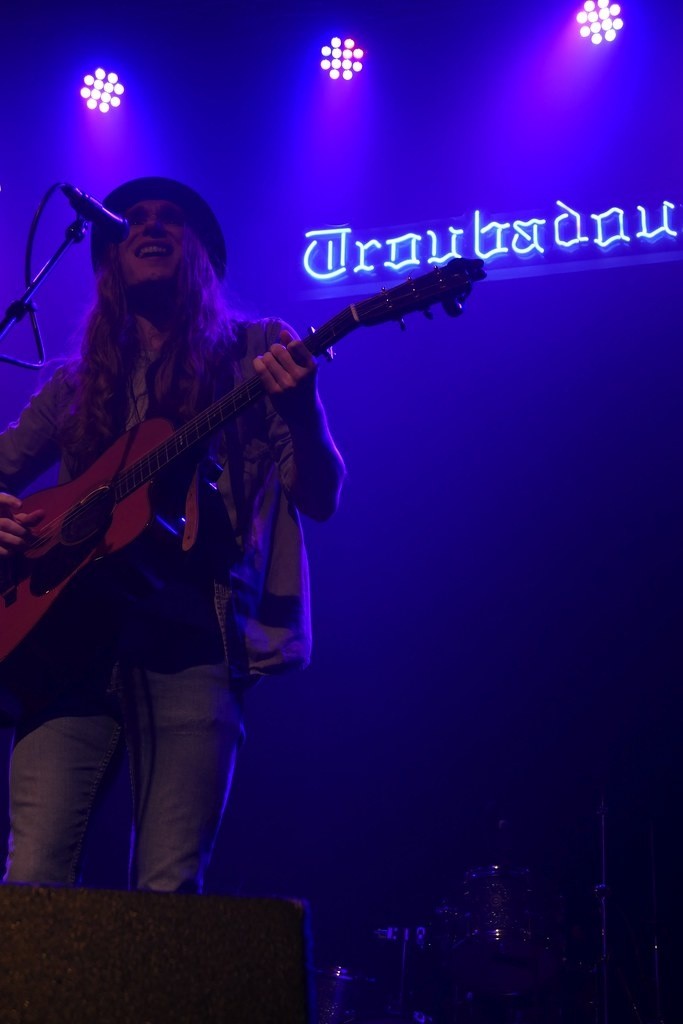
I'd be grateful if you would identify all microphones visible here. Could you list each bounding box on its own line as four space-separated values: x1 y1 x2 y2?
61 182 130 245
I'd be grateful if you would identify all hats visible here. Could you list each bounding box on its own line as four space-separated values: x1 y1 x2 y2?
91 176 226 280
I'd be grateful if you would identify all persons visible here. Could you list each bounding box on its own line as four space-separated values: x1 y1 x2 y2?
0 176 347 896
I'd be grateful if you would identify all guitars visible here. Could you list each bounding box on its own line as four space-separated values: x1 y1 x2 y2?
0 255 485 660
